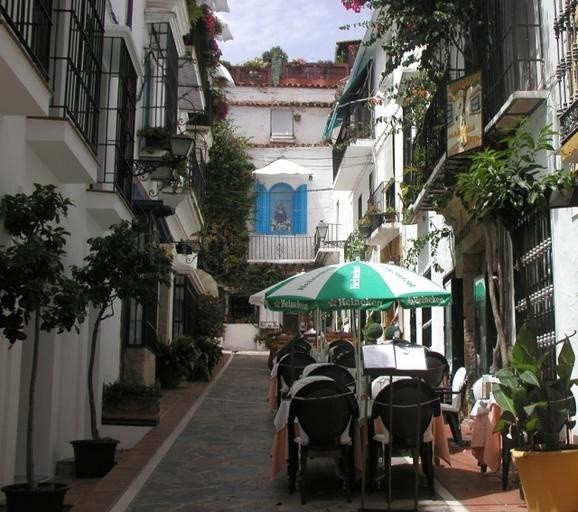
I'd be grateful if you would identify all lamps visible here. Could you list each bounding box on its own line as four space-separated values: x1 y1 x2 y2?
134 131 195 176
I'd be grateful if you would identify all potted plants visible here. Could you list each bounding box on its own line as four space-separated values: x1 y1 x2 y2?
3 181 94 504
491 320 578 511
64 205 174 474
101 368 161 426
139 318 186 389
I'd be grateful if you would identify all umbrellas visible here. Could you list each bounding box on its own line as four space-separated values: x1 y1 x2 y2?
249 267 321 336
265 255 452 400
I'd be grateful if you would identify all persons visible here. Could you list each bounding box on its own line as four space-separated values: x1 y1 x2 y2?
274 202 287 227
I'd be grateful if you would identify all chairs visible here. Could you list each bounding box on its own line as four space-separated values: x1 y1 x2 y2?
269 338 473 504
